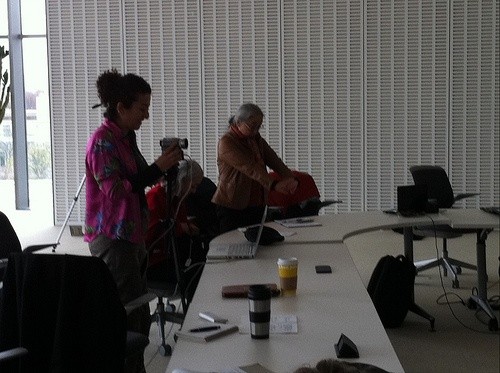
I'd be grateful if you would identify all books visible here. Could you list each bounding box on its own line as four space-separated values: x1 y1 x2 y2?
175 323 238 343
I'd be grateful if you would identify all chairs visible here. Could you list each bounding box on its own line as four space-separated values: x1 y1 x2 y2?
269 170 322 218
0 178 236 373
409 164 488 289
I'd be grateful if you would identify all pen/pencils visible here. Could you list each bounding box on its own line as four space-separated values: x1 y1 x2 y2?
187 325 221 332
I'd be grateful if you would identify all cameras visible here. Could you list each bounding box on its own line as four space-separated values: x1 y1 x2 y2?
159 137 188 154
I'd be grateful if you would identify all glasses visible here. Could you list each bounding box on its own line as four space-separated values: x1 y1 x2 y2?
241 120 263 131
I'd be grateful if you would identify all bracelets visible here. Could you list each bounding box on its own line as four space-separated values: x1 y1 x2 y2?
271 181 279 192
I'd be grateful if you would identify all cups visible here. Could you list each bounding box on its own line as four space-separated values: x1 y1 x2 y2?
277 257 298 296
247 284 271 338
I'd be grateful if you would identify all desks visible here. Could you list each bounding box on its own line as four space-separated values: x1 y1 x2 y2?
164 207 499 373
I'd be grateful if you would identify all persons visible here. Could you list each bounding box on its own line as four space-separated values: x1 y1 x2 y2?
85 67 210 340
214 104 300 234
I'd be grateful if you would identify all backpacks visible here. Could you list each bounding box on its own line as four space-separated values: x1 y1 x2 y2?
365 254 416 327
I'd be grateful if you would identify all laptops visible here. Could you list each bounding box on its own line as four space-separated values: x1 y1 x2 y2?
397 185 440 215
207 204 267 259
